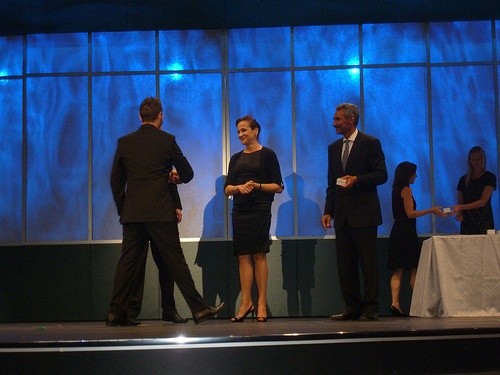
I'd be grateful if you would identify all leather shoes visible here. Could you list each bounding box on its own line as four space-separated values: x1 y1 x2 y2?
330 308 381 321
106 311 142 327
162 309 189 323
192 302 226 324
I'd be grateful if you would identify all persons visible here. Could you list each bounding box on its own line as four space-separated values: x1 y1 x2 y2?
450 146 498 234
223 114 285 323
104 97 226 328
387 161 448 317
321 103 390 321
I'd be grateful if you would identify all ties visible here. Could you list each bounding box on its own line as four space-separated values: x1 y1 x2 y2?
341 140 351 169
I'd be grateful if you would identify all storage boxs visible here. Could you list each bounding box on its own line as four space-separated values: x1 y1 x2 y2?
336 178 348 186
443 208 451 213
487 230 495 235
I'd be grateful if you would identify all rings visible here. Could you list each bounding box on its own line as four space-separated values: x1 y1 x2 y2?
246 189 248 191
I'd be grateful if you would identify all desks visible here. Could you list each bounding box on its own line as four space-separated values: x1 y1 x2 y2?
423 235 500 317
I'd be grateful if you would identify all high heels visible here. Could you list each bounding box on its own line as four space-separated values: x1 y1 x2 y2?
231 302 269 322
390 304 409 317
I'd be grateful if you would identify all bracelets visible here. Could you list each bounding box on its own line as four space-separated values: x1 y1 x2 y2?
259 183 261 191
